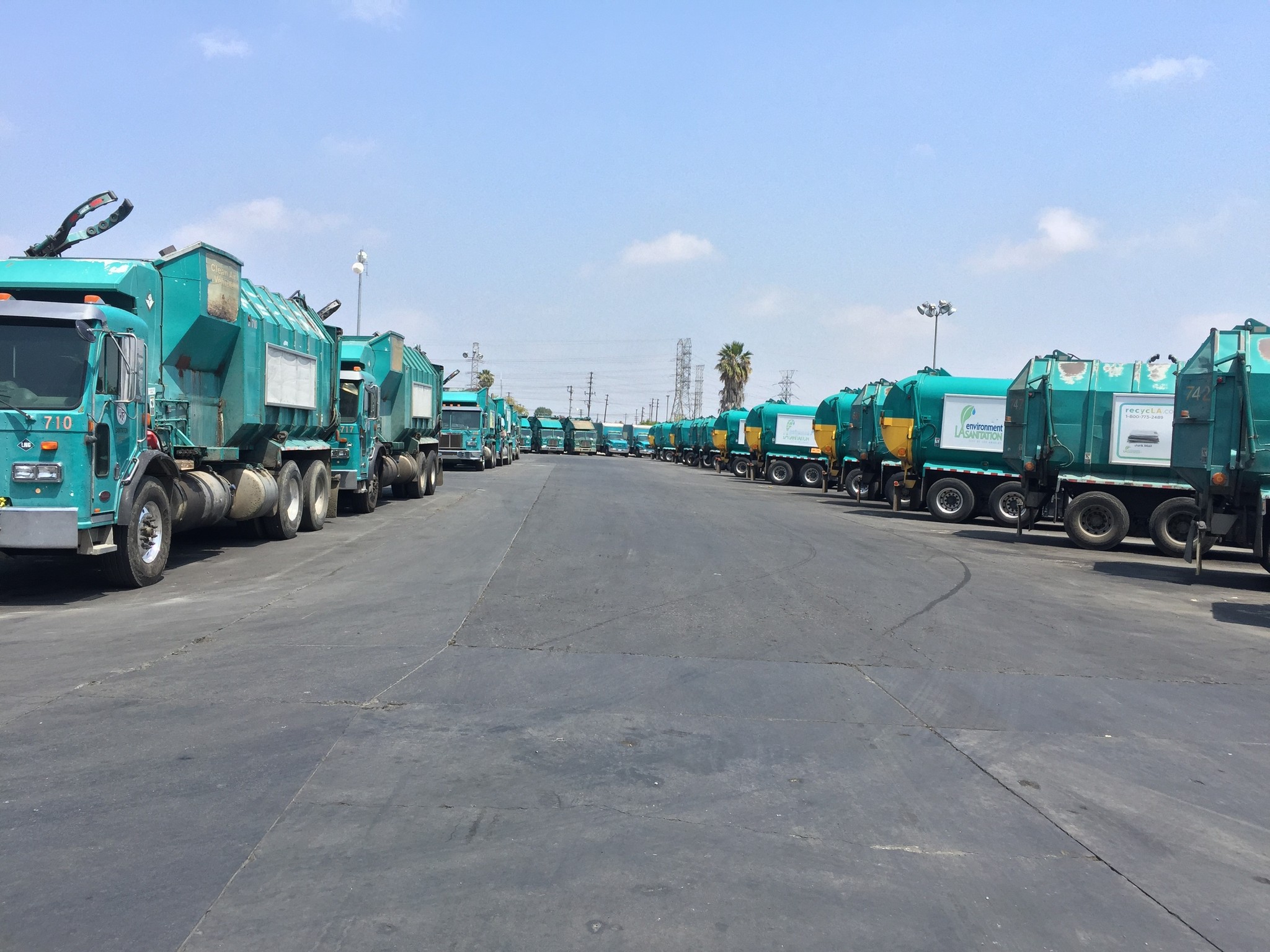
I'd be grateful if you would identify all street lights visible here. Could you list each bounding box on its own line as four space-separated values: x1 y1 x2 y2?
351 249 367 336
917 298 957 371
463 350 484 390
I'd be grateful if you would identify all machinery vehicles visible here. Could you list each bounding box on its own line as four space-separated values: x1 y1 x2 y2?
332 330 460 514
811 366 1044 530
647 399 847 489
528 414 597 456
439 386 533 472
624 424 656 460
1 188 344 589
593 422 630 457
1001 317 1270 571
